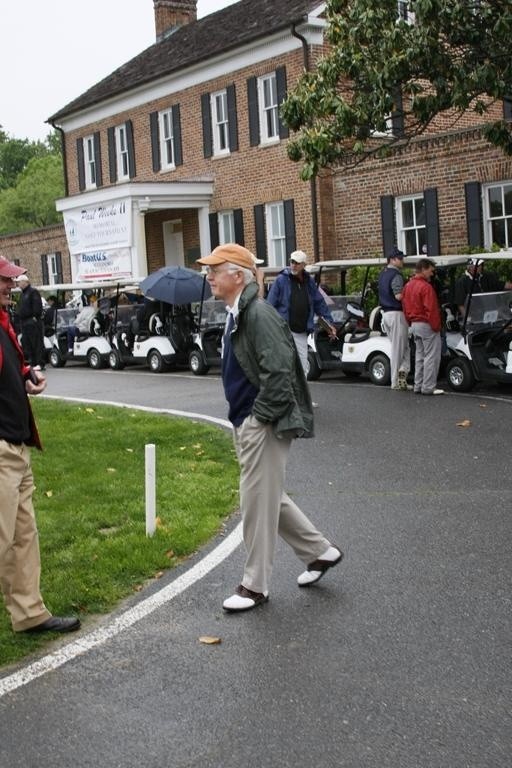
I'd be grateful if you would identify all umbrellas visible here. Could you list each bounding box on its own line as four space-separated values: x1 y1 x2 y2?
140 264 212 316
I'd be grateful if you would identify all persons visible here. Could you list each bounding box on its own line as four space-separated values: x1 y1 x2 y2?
195 244 346 612
454 256 511 332
11 273 194 373
0 254 82 634
377 248 411 391
398 258 447 396
266 249 341 384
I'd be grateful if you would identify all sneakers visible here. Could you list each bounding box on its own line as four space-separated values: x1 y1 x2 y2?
392 368 445 395
26 364 46 370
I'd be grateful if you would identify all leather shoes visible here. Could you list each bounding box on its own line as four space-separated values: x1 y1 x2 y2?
25 617 80 633
223 585 269 610
297 543 343 586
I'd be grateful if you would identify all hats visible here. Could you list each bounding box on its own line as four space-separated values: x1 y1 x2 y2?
288 250 307 263
195 243 264 276
387 248 409 257
0 255 29 282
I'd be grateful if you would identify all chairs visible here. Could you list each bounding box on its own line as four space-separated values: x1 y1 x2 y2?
344 306 388 342
